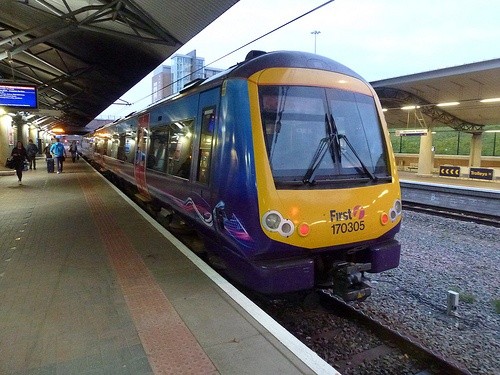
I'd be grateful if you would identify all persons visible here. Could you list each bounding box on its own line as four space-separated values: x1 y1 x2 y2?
11 140 26 184
43 143 52 161
50 138 66 174
70 140 77 163
26 139 38 170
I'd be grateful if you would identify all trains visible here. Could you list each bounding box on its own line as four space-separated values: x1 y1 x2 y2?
72 49 404 305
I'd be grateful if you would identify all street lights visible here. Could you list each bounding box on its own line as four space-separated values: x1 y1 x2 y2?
311 31 321 54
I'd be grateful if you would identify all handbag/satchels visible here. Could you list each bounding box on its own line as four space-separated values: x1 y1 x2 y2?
27 151 33 158
62 155 65 161
5 158 18 170
77 154 79 160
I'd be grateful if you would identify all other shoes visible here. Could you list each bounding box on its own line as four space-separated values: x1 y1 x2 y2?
19 181 21 185
57 171 60 174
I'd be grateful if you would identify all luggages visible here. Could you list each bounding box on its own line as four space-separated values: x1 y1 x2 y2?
47 157 54 173
